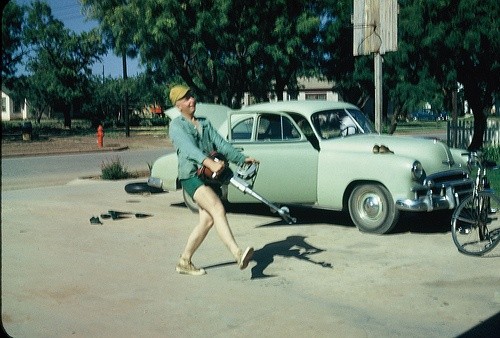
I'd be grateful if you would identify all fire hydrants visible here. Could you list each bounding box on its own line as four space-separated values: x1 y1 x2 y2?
96 124 105 149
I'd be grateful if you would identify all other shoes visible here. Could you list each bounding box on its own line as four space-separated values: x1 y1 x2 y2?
236 246 254 270
175 255 206 276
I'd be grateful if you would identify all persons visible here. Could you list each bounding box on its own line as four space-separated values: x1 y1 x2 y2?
170 86 260 275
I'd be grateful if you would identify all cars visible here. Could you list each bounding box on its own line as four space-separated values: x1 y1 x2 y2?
146 99 474 235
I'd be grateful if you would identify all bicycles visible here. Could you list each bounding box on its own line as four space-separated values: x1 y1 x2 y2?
450 151 500 256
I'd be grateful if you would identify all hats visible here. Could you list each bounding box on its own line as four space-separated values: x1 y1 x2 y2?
169 85 192 106
